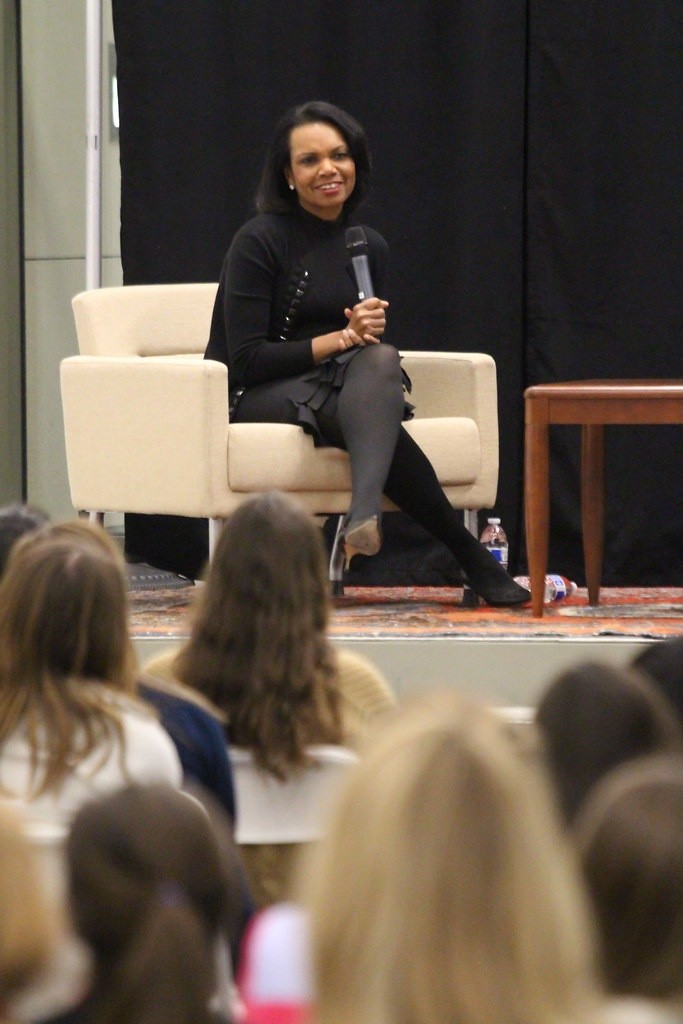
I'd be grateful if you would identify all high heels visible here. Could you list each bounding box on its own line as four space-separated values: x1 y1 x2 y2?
334 497 383 573
458 557 531 609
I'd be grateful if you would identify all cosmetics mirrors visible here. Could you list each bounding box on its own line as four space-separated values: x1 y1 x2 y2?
239 901 317 1008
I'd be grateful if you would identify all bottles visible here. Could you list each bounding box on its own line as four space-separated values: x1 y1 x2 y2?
480 517 509 571
511 574 579 606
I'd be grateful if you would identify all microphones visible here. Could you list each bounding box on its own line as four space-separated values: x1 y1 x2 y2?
345 227 374 303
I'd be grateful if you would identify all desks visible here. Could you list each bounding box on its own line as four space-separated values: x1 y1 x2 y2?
523 378 683 619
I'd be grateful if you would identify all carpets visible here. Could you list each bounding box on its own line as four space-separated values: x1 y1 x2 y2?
114 587 683 640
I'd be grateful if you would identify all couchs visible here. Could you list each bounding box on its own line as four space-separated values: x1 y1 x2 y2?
59 282 502 604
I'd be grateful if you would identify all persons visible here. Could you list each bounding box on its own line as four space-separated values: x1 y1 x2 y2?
144 492 400 764
30 780 266 1024
533 634 682 1024
1 503 256 1024
205 103 533 611
304 697 608 1024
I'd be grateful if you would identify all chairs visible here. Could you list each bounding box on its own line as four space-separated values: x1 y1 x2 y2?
222 741 364 848
